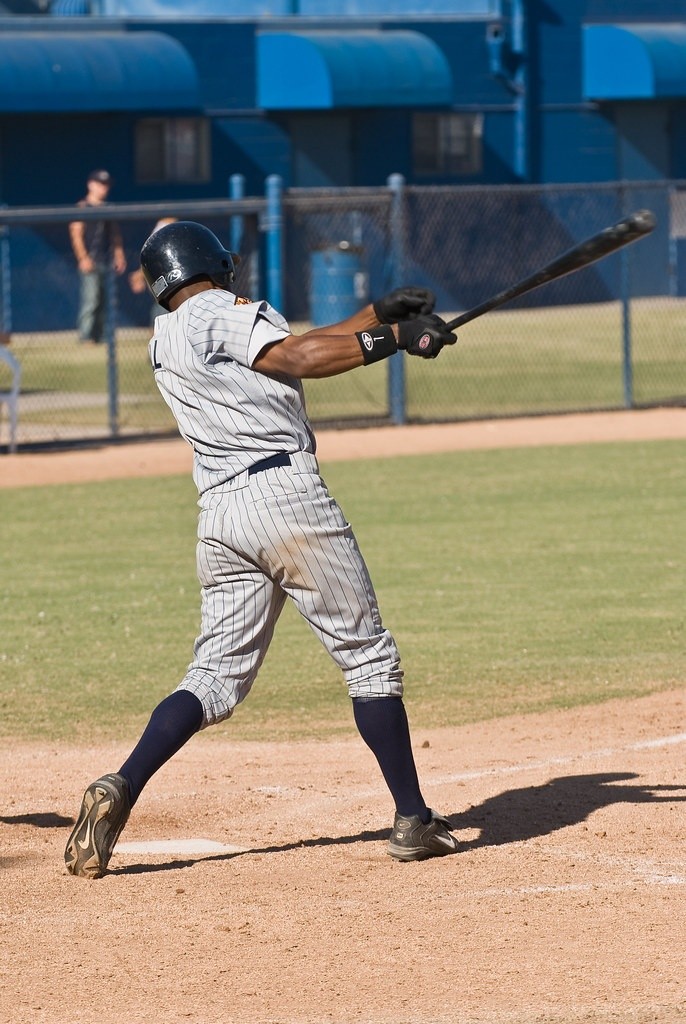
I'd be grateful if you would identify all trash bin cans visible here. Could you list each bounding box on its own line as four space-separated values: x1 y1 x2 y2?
307 243 361 330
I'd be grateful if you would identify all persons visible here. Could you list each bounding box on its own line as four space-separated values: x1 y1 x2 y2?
63 222 462 881
131 218 178 292
68 170 126 344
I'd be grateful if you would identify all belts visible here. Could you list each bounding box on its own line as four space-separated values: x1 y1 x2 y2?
249 454 291 476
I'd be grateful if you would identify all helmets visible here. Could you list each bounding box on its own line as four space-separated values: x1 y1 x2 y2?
139 221 240 312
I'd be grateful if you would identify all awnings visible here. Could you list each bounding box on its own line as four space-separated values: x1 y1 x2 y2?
1 31 205 112
581 21 686 99
256 28 454 109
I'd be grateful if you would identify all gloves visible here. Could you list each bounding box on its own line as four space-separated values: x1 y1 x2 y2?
397 314 458 359
373 286 436 326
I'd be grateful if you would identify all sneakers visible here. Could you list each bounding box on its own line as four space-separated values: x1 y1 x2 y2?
64 772 131 879
387 807 460 861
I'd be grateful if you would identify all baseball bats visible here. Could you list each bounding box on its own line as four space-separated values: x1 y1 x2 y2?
446 210 658 331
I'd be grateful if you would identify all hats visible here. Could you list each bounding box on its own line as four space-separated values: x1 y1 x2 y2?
88 171 110 184
